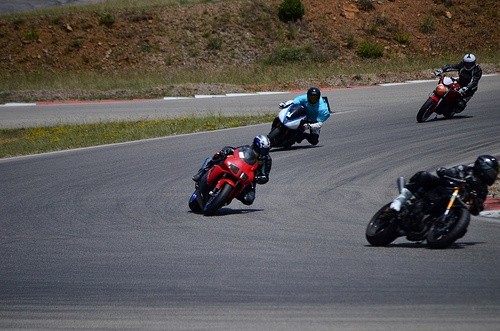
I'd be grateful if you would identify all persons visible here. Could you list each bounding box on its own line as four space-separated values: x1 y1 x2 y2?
279 87 331 144
432 53 482 106
422 154 499 223
192 135 272 205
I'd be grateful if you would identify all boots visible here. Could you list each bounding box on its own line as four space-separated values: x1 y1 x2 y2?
192 168 208 182
389 187 412 212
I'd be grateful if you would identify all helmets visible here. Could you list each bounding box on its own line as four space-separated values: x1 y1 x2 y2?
252 135 270 156
307 88 321 106
463 54 476 71
473 154 499 187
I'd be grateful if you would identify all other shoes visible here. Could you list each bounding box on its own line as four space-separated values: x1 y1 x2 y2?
296 135 306 143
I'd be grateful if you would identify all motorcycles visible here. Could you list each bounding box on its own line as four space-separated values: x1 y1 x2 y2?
416 72 468 123
188 144 260 216
268 96 335 152
366 165 477 250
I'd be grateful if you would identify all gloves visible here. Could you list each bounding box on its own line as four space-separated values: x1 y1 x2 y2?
303 123 313 130
469 203 485 217
433 67 444 77
278 102 287 109
458 86 470 97
444 168 461 187
255 174 268 184
223 147 235 155
